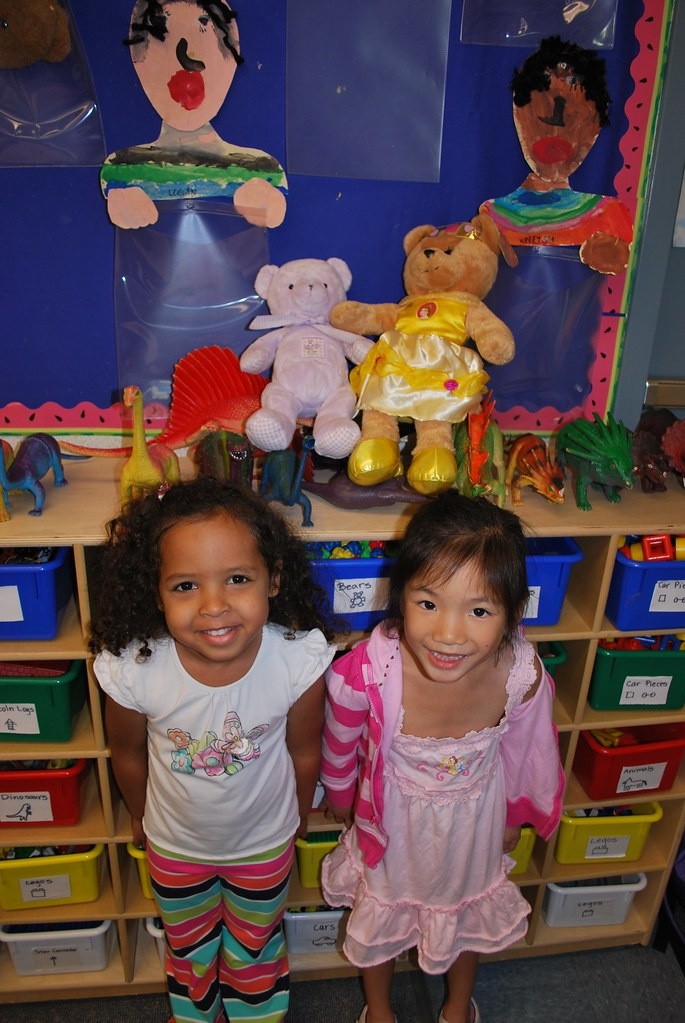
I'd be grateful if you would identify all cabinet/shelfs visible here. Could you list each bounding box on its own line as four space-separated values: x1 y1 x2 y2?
0 459 685 1005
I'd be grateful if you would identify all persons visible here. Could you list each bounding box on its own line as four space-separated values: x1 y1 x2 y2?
85 476 337 1023
320 492 565 1023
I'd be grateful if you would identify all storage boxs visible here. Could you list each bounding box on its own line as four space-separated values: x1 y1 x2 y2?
127 842 155 901
0 660 88 743
0 547 72 642
145 917 167 971
604 549 685 632
282 908 352 956
0 844 107 912
586 645 685 712
0 758 91 829
572 722 685 801
0 919 118 975
541 871 648 928
537 641 568 674
555 801 665 866
307 557 398 633
295 838 339 890
505 827 538 877
515 537 584 627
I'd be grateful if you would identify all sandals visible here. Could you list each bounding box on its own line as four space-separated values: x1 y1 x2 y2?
439 996 480 1023
356 1004 397 1023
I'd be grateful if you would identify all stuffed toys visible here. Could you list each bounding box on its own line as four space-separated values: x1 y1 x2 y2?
239 256 377 459
329 215 514 494
